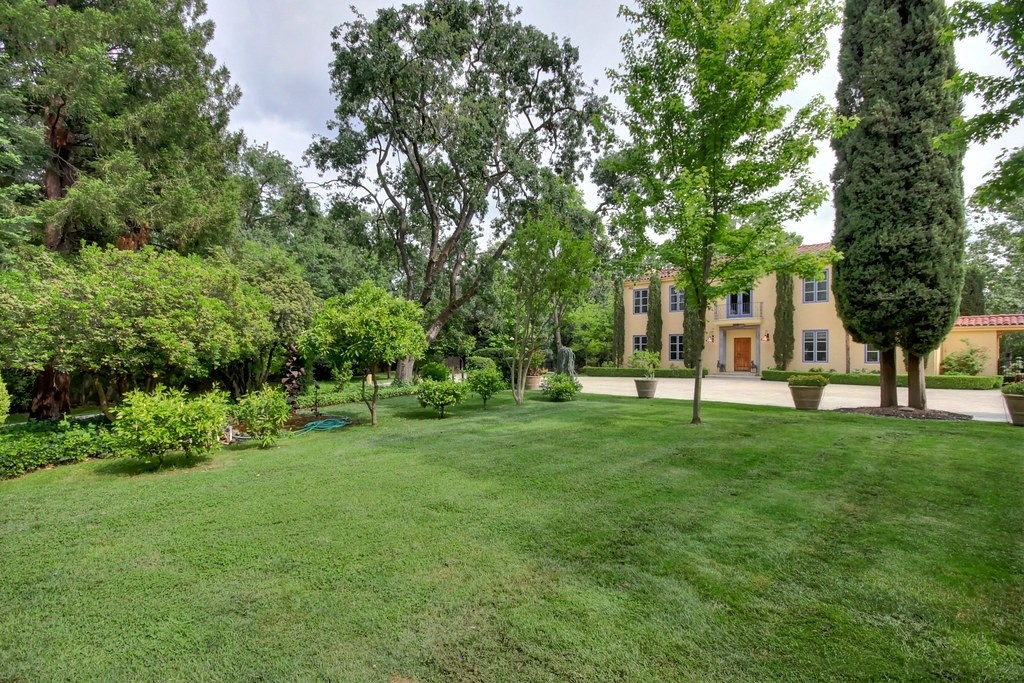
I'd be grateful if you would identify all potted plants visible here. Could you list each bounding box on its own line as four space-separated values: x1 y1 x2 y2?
787 374 830 410
526 349 546 390
635 368 658 398
1002 380 1024 426
